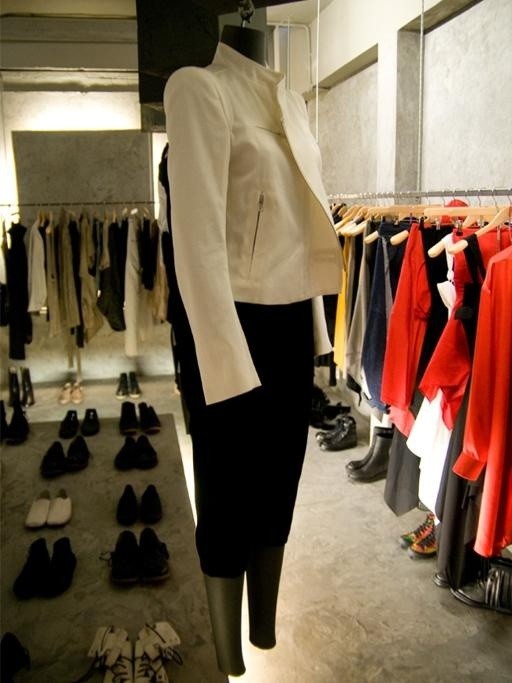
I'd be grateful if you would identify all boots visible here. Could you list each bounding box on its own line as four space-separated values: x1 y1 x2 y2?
399 513 512 619
59 382 83 406
114 371 141 399
314 411 354 453
12 536 77 602
119 400 163 436
89 619 182 682
1 398 30 446
345 420 396 483
6 364 35 407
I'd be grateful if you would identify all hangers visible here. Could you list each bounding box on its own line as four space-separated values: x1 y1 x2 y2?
323 185 511 259
1 195 157 235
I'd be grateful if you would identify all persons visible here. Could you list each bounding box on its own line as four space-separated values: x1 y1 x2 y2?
162 25 345 677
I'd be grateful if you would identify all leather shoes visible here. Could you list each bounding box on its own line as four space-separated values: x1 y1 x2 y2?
23 486 73 530
114 433 158 473
59 408 101 441
108 529 171 593
117 482 164 528
40 433 91 479
